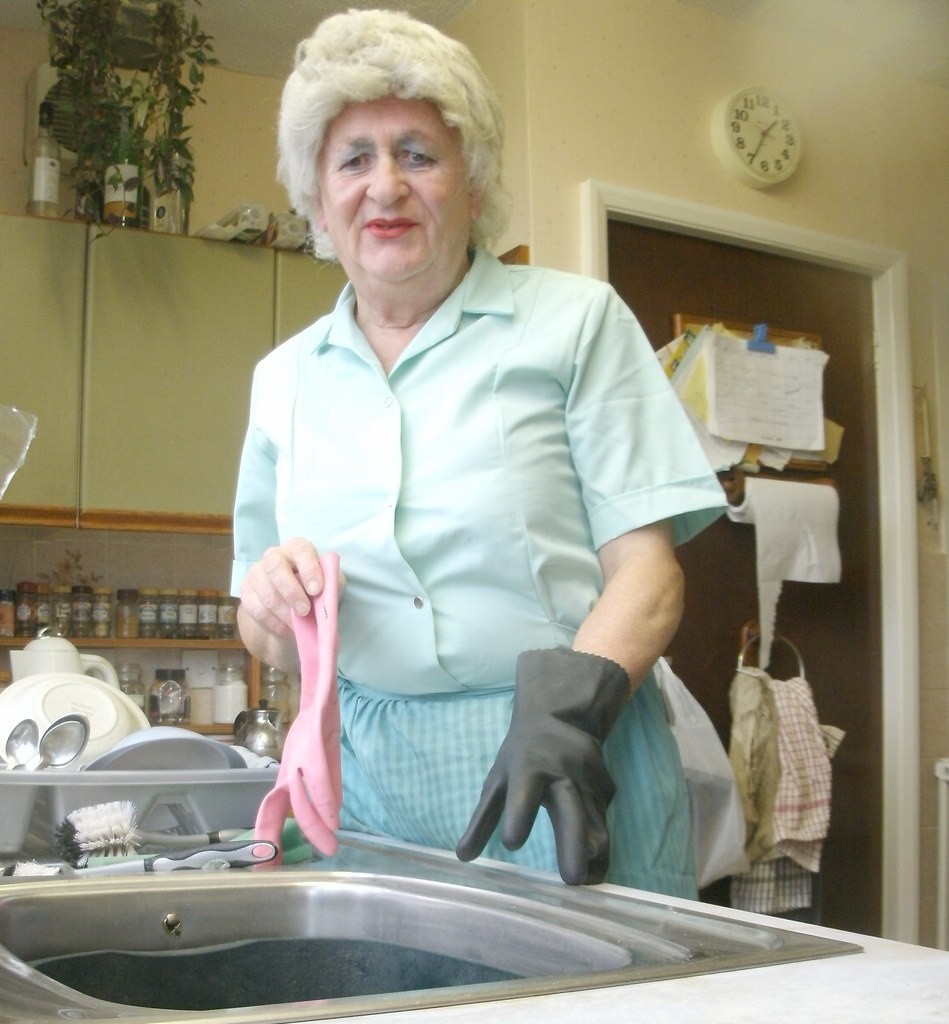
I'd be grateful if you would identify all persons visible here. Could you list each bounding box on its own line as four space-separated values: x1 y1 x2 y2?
227 6 738 908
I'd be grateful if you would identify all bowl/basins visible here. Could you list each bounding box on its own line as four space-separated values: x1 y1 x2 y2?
229 744 280 770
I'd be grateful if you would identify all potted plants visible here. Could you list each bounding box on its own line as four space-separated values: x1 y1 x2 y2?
36 0 223 251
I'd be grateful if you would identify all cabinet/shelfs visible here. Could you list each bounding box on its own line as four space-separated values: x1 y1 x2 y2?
0 217 89 528
80 220 350 534
0 636 260 734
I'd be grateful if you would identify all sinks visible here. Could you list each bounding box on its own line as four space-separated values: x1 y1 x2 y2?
0 839 868 1024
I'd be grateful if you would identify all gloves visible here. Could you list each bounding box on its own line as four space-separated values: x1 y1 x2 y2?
256 552 343 868
456 649 630 885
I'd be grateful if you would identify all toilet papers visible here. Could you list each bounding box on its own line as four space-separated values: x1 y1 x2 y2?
725 477 843 671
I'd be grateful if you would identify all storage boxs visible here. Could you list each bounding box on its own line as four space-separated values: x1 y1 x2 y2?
0 767 278 857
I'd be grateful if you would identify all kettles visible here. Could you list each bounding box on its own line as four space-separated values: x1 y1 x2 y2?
232 699 287 762
9 625 121 690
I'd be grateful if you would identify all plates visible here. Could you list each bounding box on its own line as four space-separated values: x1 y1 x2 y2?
0 671 250 772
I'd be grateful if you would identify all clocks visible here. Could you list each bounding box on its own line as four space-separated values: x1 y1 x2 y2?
711 84 807 190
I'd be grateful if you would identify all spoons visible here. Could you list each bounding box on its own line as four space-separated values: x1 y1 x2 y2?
1 712 91 771
